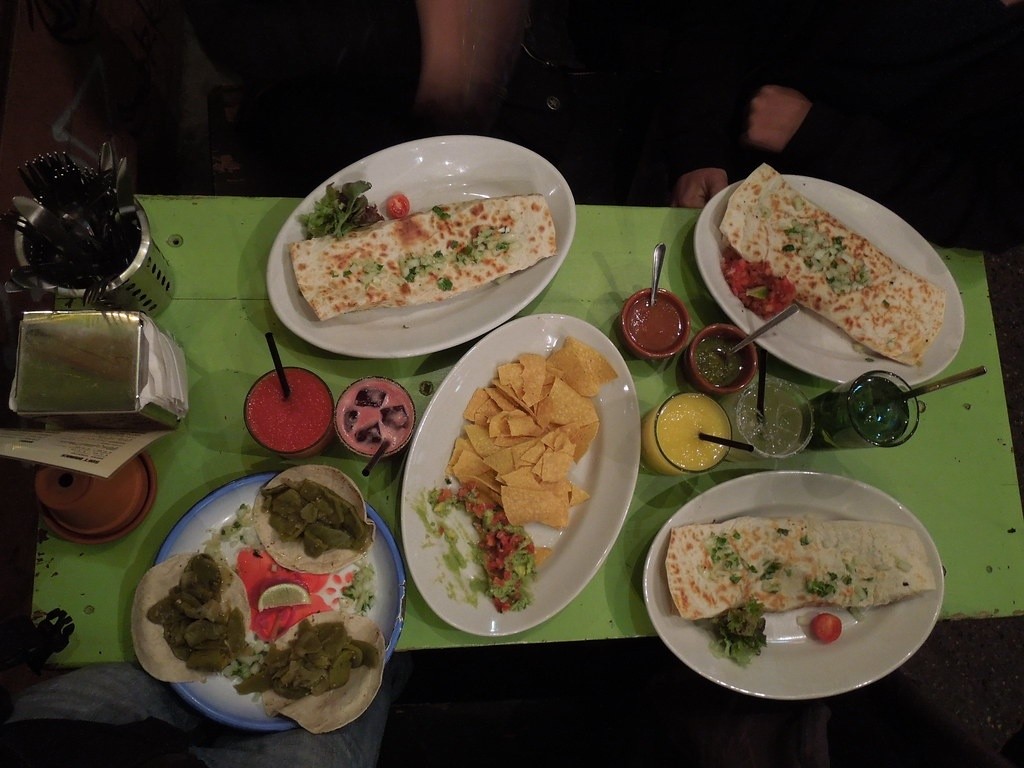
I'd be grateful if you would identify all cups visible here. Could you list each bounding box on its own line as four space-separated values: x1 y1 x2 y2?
242 366 334 457
717 372 814 465
619 289 689 360
638 392 732 475
684 324 760 396
333 374 416 458
806 370 920 451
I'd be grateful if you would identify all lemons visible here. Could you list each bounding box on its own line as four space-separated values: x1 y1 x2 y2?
257 583 311 610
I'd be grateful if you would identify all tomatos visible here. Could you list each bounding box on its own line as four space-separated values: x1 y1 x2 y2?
387 194 411 218
811 613 842 643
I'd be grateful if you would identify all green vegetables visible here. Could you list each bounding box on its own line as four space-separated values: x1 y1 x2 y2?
299 181 385 239
694 527 876 667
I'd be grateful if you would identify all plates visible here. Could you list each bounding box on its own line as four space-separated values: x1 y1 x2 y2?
401 313 643 637
694 174 966 391
640 469 946 698
141 471 407 730
266 135 577 356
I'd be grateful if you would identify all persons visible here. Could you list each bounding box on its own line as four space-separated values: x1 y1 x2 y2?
672 0 848 208
395 1 536 137
2 650 415 768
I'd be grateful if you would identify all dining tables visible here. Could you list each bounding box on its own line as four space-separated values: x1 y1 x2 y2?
26 194 1024 671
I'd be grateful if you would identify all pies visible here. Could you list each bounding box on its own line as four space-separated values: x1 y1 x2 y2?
666 516 937 620
260 609 385 733
289 193 559 322
719 161 946 367
129 552 250 684
252 464 375 576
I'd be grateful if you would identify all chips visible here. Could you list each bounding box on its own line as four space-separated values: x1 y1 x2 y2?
448 336 618 530
